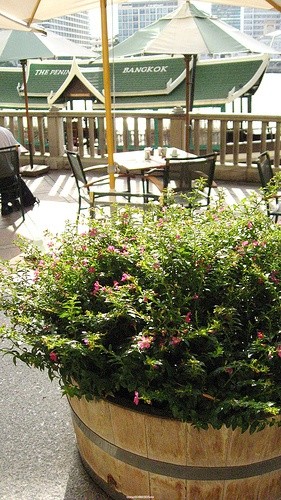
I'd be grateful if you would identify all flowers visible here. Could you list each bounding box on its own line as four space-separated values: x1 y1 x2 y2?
0 169 281 435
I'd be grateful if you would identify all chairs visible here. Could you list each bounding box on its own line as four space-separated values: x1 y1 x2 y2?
0 145 25 222
65 150 135 220
256 152 281 223
140 152 220 211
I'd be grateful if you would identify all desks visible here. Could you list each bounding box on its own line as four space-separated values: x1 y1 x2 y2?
103 147 198 203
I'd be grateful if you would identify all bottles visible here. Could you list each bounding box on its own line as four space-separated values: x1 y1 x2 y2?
144 146 178 159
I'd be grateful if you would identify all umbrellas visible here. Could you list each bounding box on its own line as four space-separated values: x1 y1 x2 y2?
89 1 281 177
0 24 100 177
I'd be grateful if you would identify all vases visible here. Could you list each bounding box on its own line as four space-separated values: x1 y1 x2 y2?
63 372 281 500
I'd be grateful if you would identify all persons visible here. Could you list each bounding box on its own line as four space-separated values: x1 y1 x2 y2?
0 125 20 177
